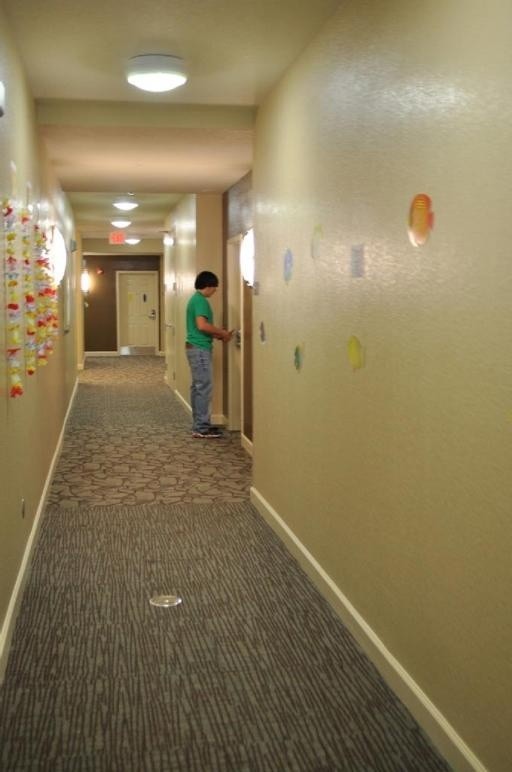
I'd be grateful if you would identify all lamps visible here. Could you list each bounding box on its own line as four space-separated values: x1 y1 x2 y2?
126 54 190 93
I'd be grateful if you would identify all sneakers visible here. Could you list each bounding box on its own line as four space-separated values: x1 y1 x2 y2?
192 425 222 438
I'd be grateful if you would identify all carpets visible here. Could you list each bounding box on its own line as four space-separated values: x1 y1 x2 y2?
66 381 192 425
2 502 461 772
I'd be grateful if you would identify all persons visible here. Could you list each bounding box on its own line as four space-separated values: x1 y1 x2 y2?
184 271 234 439
